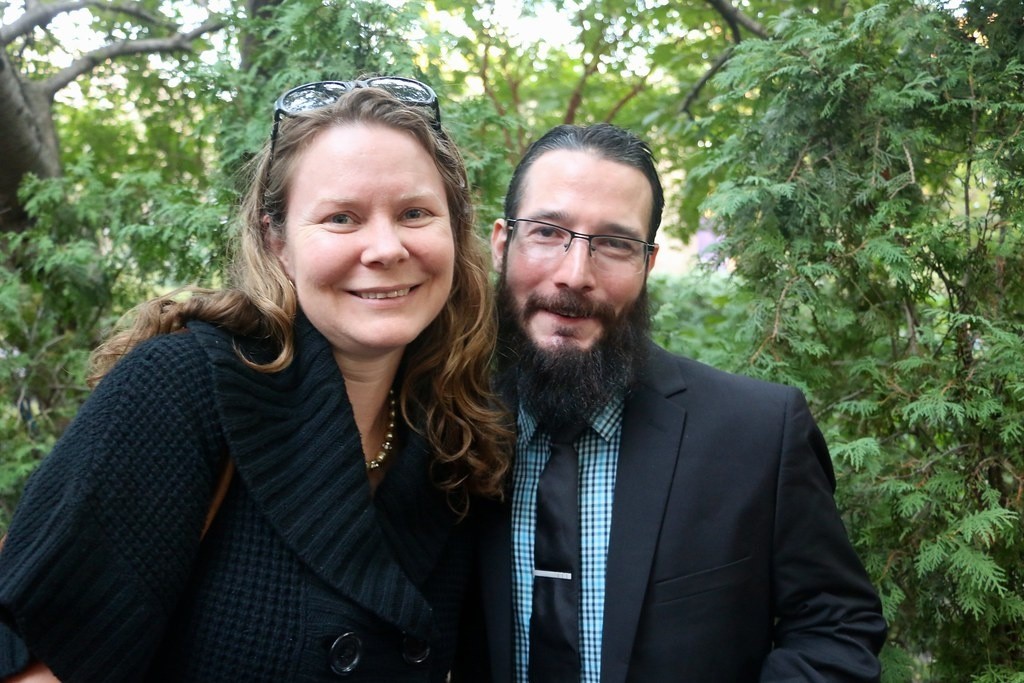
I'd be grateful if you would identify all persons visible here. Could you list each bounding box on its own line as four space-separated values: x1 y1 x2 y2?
454 123 889 683
0 76 516 683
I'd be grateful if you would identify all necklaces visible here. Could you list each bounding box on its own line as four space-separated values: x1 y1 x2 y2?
365 388 396 471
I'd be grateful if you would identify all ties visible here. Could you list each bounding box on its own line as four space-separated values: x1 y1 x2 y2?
526 412 602 683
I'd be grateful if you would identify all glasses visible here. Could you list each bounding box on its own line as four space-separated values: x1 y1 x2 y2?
266 76 441 191
506 219 654 274
